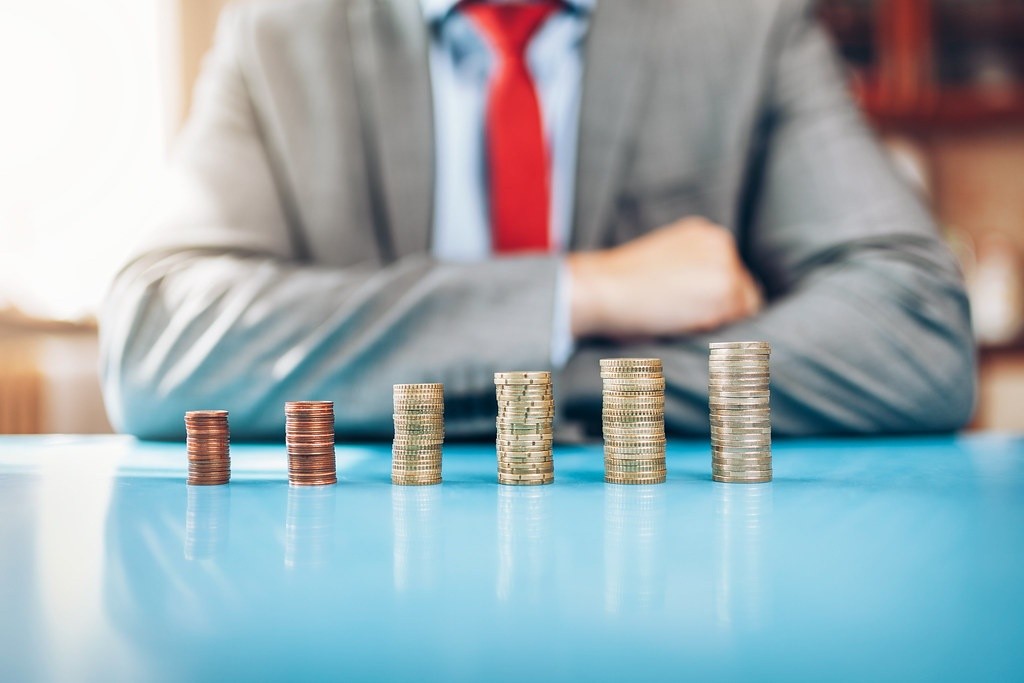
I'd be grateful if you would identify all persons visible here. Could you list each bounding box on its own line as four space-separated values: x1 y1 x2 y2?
98 0 978 442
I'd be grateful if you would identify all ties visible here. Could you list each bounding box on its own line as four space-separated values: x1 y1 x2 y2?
458 1 565 253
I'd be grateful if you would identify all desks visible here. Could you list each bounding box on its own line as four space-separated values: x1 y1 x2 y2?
0 433 1024 683
